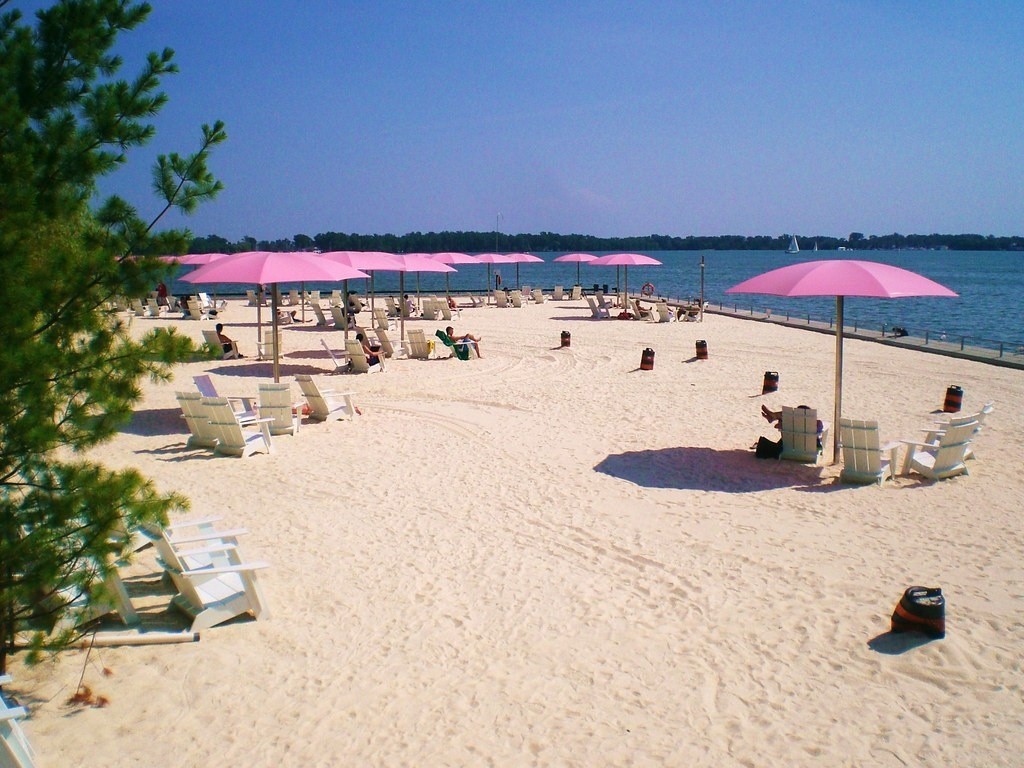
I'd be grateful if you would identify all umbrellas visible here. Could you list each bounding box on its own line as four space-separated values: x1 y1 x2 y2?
724 260 960 465
256 252 665 362
112 251 201 298
183 254 231 310
178 246 371 381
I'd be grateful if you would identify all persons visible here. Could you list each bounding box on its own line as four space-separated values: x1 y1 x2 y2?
178 295 220 318
446 295 458 311
277 307 313 323
156 279 167 307
387 296 401 317
656 299 674 320
215 323 245 358
348 291 366 308
334 306 361 327
501 287 512 303
355 334 385 372
760 404 825 439
403 294 416 313
678 301 704 322
635 299 654 320
446 326 485 359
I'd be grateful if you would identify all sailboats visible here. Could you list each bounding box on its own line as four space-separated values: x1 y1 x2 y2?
784 233 800 254
812 241 818 252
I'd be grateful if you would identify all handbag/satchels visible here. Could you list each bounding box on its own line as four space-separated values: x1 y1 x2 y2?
750 436 779 460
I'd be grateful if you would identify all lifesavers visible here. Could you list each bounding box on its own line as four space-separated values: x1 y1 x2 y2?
642 284 654 296
499 276 501 285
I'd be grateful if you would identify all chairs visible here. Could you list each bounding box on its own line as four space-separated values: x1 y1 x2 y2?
0 676 37 768
778 406 830 464
113 290 709 633
934 399 996 460
899 412 980 484
838 417 902 485
